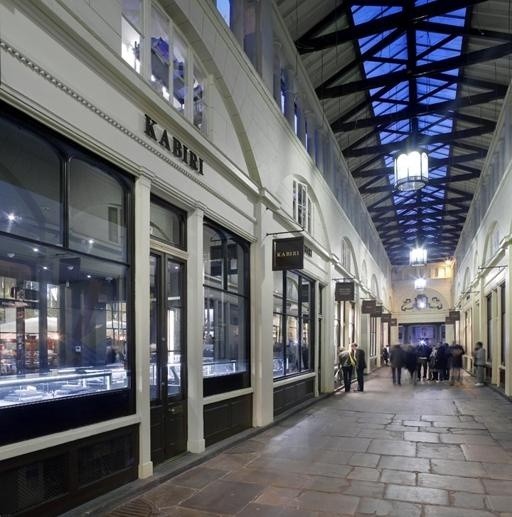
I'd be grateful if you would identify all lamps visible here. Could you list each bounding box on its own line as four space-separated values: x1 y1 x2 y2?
409 246 428 266
392 143 429 191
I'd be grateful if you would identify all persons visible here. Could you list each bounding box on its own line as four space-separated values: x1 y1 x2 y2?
383 341 465 385
350 342 367 391
472 341 486 385
338 347 353 391
106 336 116 362
273 339 308 368
